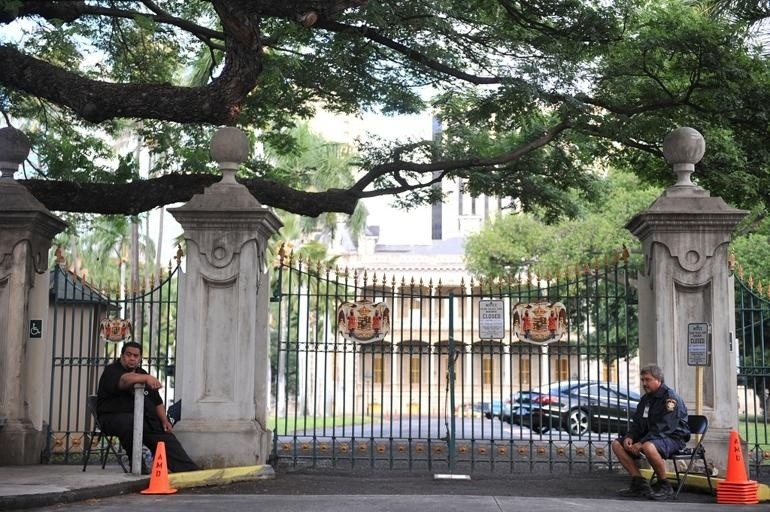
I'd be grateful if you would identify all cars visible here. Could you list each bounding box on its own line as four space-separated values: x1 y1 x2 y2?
505 377 641 441
475 396 512 424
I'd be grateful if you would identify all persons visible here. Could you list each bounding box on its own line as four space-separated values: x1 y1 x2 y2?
346 308 357 338
612 363 691 500
381 307 390 339
559 308 566 335
97 342 199 475
338 309 346 336
99 320 132 340
513 310 520 337
372 309 381 339
547 309 559 340
520 309 533 339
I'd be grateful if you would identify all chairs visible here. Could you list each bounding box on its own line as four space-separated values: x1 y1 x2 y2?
646 415 715 499
81 394 134 473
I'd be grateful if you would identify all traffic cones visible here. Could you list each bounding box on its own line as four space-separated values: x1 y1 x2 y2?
714 426 759 505
138 439 187 495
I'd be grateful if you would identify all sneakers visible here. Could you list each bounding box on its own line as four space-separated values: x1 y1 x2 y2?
646 477 676 501
130 464 152 478
615 475 647 497
